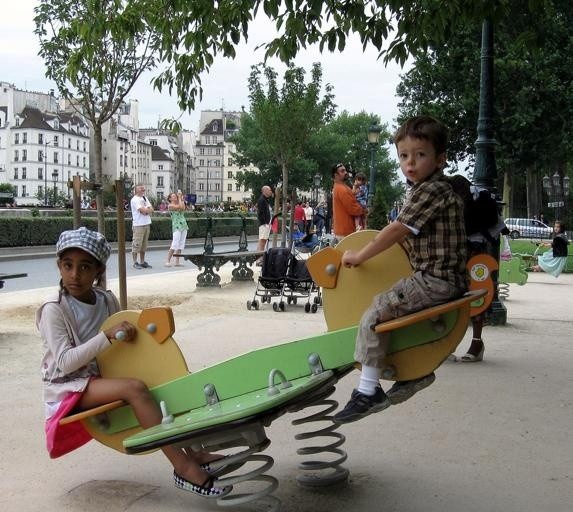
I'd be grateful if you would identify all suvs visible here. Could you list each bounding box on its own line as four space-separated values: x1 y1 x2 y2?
503 217 568 240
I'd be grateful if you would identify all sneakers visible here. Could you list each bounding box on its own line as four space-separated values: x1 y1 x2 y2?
174 263 183 267
200 455 248 477
140 261 151 269
172 468 232 497
132 262 143 269
164 262 172 266
331 382 390 423
256 261 261 265
385 373 435 405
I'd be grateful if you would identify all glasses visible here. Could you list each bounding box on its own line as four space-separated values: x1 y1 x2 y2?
334 163 343 168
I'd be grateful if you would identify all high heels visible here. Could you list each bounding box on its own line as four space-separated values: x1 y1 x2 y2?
446 354 457 362
461 338 484 361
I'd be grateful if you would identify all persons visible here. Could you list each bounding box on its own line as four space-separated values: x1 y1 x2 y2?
165 192 189 267
315 200 325 234
122 193 128 210
304 201 313 235
35 226 232 498
532 221 570 278
295 201 307 234
533 214 539 226
333 116 472 424
130 185 154 269
89 199 97 209
352 173 369 232
453 175 500 363
257 185 273 265
302 203 305 207
158 199 168 210
64 196 73 208
329 164 369 242
390 204 397 221
538 213 549 225
294 201 299 207
81 196 89 209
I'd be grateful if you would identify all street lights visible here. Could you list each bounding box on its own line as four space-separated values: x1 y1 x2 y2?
314 174 320 206
366 120 382 207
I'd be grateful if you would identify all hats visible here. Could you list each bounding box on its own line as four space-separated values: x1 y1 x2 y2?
54 227 110 266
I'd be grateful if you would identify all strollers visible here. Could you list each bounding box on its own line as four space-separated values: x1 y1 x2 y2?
247 211 327 313
